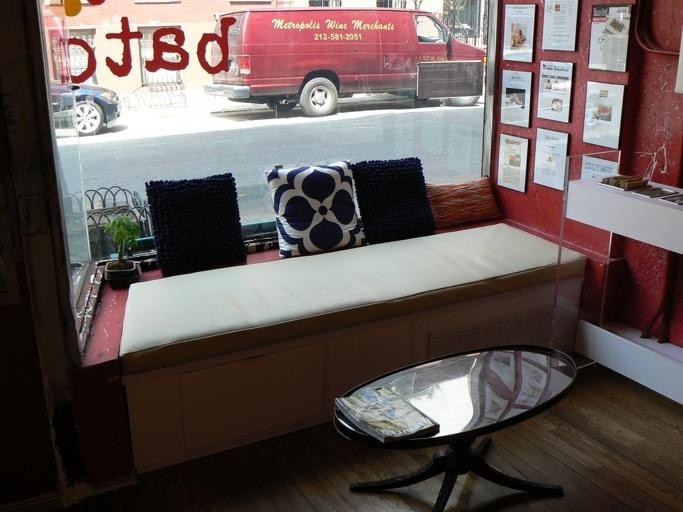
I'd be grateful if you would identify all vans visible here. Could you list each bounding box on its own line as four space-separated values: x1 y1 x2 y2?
203 6 486 117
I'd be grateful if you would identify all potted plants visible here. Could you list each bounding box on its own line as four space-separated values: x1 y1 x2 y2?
105 213 142 289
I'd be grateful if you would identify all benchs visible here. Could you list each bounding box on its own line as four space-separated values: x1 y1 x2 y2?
118 225 586 474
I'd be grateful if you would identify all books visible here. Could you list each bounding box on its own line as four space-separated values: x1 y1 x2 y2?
658 193 683 206
333 385 440 447
633 187 680 198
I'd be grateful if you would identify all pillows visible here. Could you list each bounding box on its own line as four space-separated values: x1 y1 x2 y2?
355 159 436 246
145 172 247 276
264 161 368 259
427 176 503 228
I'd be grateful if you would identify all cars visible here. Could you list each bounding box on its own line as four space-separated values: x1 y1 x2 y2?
49 82 123 136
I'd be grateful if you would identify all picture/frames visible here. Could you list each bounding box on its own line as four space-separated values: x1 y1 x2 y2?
497 4 633 194
